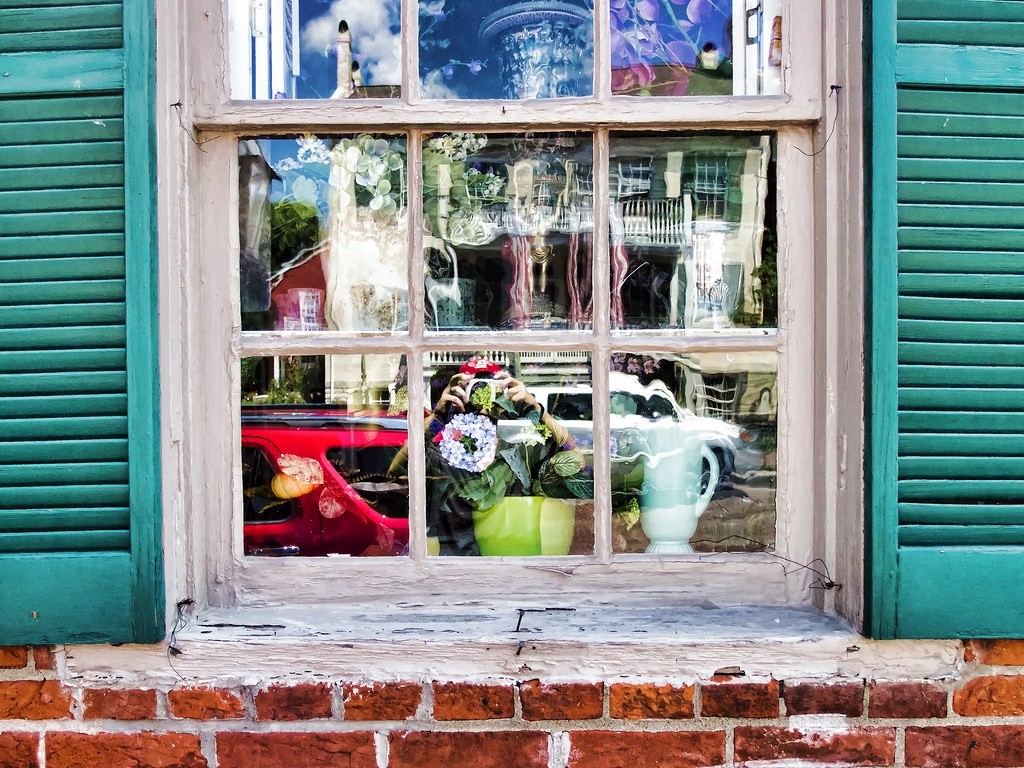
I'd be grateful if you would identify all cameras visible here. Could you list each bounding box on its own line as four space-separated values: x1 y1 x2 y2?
464 379 509 402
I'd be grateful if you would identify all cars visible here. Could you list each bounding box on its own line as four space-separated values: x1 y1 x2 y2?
243 406 464 563
527 386 767 501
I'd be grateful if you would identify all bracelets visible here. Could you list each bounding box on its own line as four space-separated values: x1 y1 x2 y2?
536 403 547 419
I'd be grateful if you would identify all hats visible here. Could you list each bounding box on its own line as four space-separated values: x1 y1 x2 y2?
459 359 500 378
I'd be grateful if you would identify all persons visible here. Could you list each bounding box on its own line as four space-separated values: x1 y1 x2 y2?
387 355 577 562
